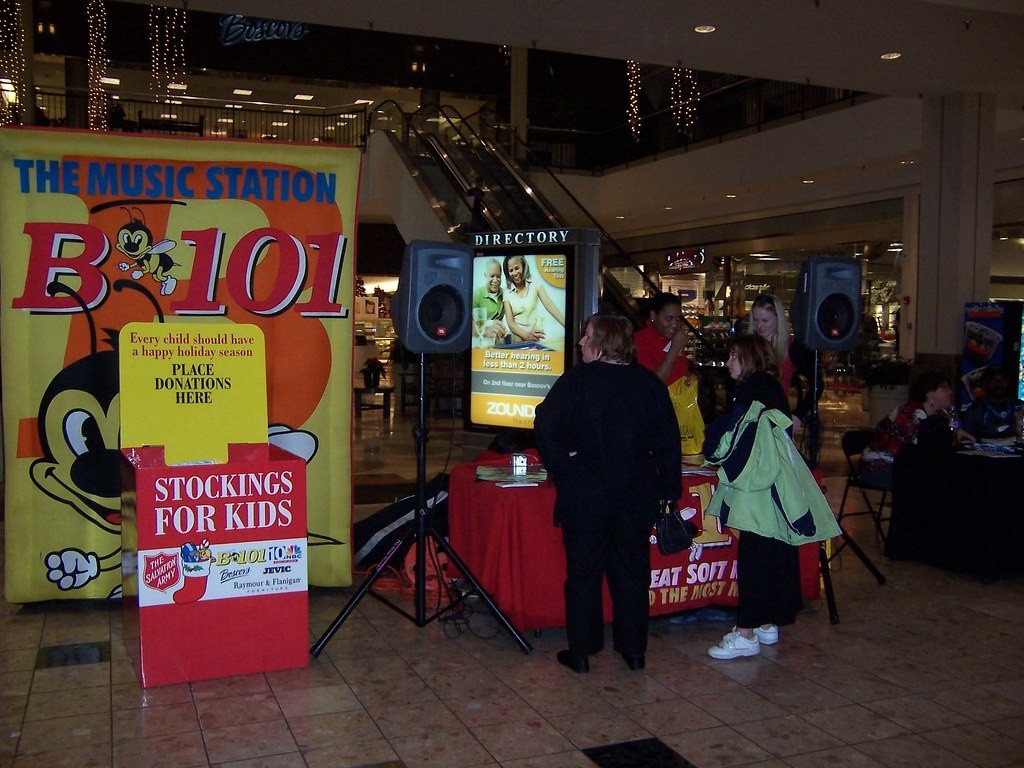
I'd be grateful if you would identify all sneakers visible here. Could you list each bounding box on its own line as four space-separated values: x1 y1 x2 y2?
707 626 760 660
754 626 778 646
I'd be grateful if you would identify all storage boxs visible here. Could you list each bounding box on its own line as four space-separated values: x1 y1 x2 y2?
119 443 309 690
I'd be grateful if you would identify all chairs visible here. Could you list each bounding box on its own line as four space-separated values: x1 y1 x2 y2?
837 429 894 544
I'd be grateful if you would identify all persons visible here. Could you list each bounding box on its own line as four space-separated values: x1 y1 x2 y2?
635 292 689 386
857 372 976 492
472 255 565 347
533 311 682 674
961 366 1024 440
702 294 842 661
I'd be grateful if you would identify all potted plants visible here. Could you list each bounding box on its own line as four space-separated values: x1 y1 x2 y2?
358 357 388 388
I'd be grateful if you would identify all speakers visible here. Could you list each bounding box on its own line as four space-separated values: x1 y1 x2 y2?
391 240 474 355
789 256 862 351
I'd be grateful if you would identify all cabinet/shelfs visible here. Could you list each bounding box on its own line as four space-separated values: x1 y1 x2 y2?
354 316 395 370
663 280 732 368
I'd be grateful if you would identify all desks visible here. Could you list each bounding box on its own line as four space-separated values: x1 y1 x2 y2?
354 384 395 417
445 448 820 639
884 443 1024 586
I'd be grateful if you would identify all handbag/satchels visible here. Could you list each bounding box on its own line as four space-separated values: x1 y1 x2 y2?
666 376 705 456
654 497 693 553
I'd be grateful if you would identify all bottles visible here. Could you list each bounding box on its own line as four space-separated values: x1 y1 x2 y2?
949 405 959 451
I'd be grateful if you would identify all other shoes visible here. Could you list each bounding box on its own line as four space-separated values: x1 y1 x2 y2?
556 650 589 673
621 652 645 671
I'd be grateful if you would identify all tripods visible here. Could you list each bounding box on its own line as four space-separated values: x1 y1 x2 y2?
311 358 535 658
808 351 887 624
